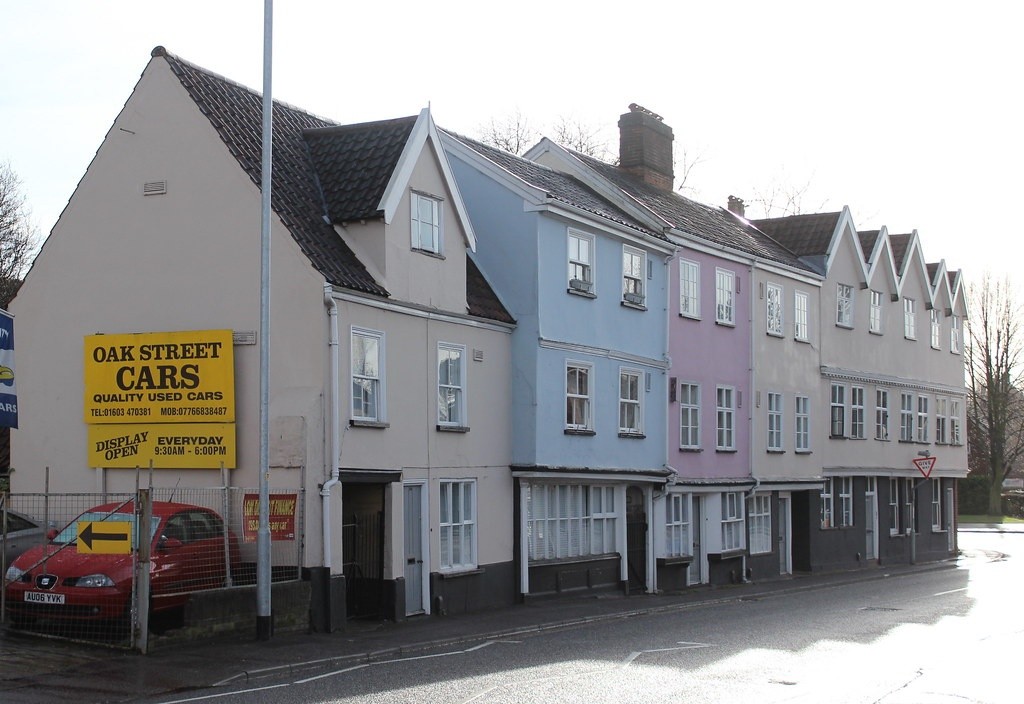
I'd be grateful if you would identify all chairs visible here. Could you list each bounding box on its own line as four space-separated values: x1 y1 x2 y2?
164 520 207 542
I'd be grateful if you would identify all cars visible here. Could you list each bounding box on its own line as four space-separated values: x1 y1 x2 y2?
5 501 242 639
0 507 57 572
